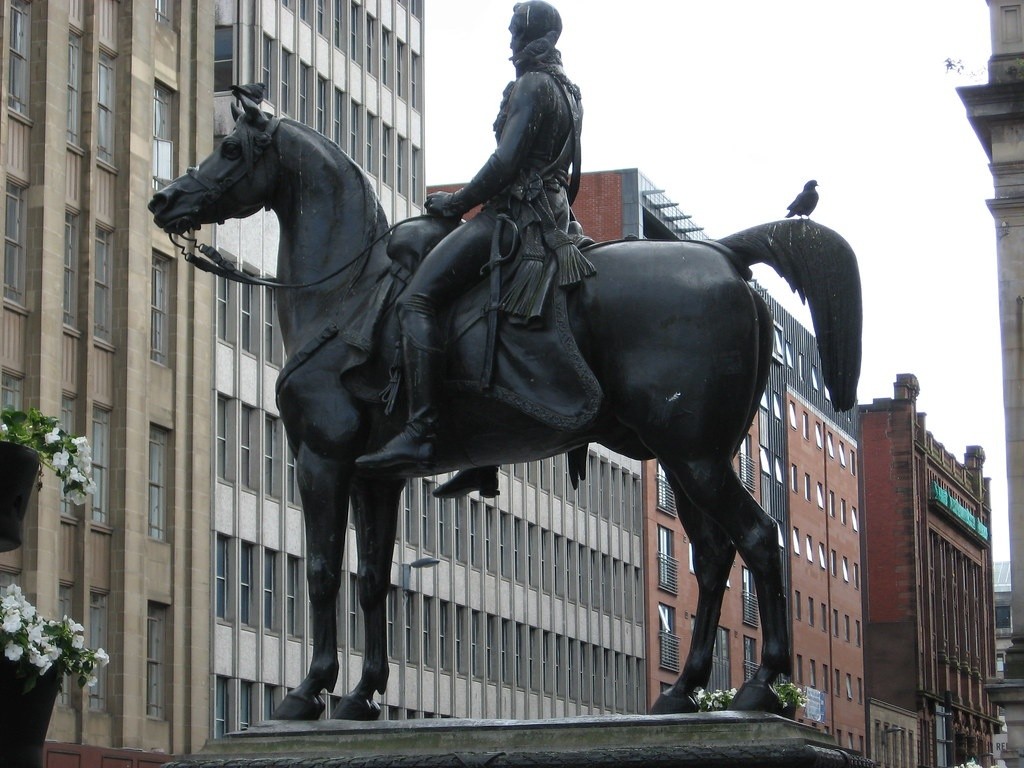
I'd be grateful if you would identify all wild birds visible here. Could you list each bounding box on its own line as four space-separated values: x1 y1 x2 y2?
230 82 266 110
785 180 819 219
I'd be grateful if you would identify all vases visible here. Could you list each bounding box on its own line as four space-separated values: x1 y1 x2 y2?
775 702 796 720
0 441 39 553
0 662 58 768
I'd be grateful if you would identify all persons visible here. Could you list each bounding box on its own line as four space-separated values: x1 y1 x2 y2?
354 0 604 467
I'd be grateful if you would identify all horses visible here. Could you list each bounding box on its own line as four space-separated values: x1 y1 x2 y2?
146 94 862 720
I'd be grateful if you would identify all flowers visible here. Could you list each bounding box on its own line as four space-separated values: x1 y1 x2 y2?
768 680 810 709
0 404 98 506
0 583 109 704
693 687 738 712
